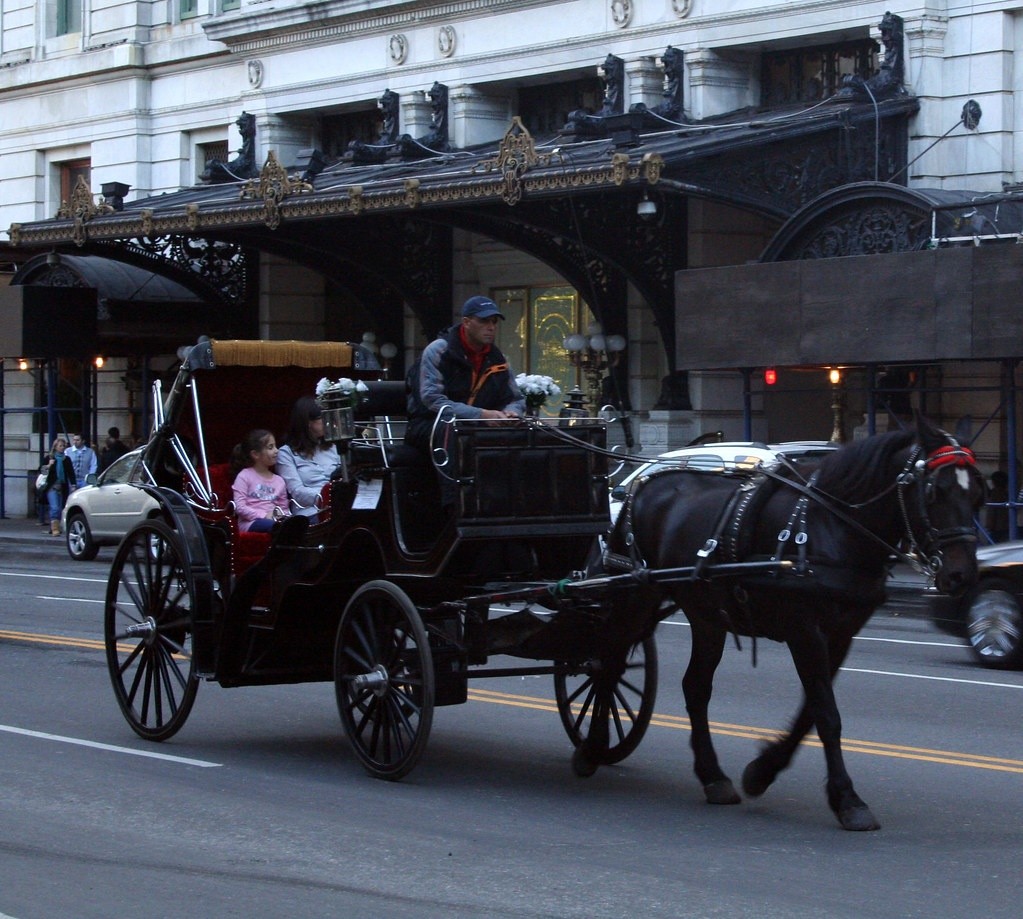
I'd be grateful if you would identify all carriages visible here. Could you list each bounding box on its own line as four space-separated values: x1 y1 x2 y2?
100 330 993 832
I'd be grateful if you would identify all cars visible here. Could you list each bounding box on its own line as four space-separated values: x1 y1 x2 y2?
59 443 177 566
923 537 1023 671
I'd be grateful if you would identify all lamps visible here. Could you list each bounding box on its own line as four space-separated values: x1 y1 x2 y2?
612 128 640 150
100 182 133 211
292 147 327 183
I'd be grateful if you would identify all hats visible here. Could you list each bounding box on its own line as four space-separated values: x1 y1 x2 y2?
461 295 507 321
109 427 120 439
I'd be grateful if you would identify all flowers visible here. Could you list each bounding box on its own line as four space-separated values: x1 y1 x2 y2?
515 373 562 408
315 377 370 409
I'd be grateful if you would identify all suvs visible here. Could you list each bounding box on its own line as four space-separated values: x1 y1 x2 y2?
597 439 845 558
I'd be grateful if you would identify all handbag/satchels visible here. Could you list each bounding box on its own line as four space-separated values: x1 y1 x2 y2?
36 474 48 492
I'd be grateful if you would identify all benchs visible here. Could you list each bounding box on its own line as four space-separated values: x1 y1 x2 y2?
181 464 271 593
347 379 419 480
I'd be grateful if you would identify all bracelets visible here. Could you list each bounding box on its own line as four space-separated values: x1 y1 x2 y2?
71 485 76 486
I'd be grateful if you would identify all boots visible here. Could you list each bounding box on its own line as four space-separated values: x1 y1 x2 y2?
51 519 60 537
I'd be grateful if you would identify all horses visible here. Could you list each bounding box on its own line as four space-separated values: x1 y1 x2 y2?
570 409 989 830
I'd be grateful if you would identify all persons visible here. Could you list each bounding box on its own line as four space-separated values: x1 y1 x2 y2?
232 429 292 533
405 296 527 517
90 427 146 475
274 398 341 524
39 438 76 536
64 434 97 494
